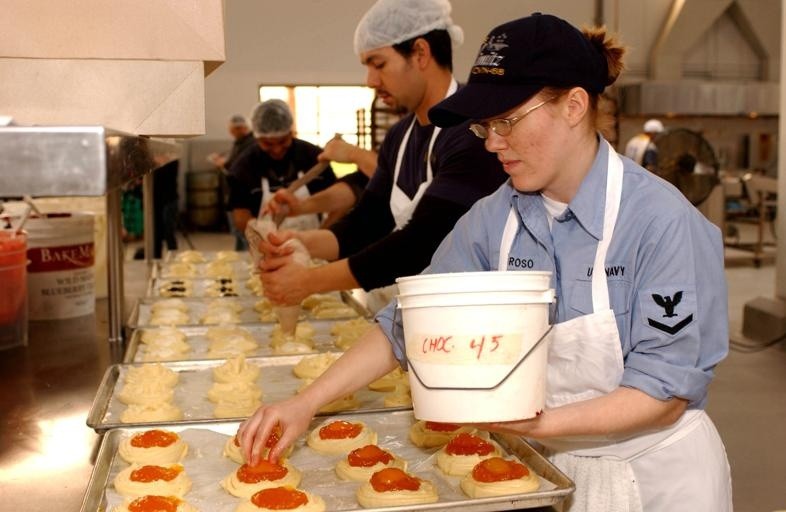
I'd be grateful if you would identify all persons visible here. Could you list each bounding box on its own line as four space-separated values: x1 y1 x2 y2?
208 114 257 251
227 99 338 252
257 0 513 306
234 12 734 511
625 119 664 174
268 135 379 229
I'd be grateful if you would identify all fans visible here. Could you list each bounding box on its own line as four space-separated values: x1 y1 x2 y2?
640 128 717 207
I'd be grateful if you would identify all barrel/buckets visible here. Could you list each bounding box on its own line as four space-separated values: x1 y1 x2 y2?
0 230 27 322
391 271 557 424
11 209 105 320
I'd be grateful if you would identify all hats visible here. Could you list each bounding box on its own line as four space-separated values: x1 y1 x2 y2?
642 119 666 133
427 12 609 128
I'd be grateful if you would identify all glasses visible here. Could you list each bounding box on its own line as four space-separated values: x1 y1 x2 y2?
469 89 572 138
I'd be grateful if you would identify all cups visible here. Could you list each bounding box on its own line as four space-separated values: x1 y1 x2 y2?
0 229 31 350
